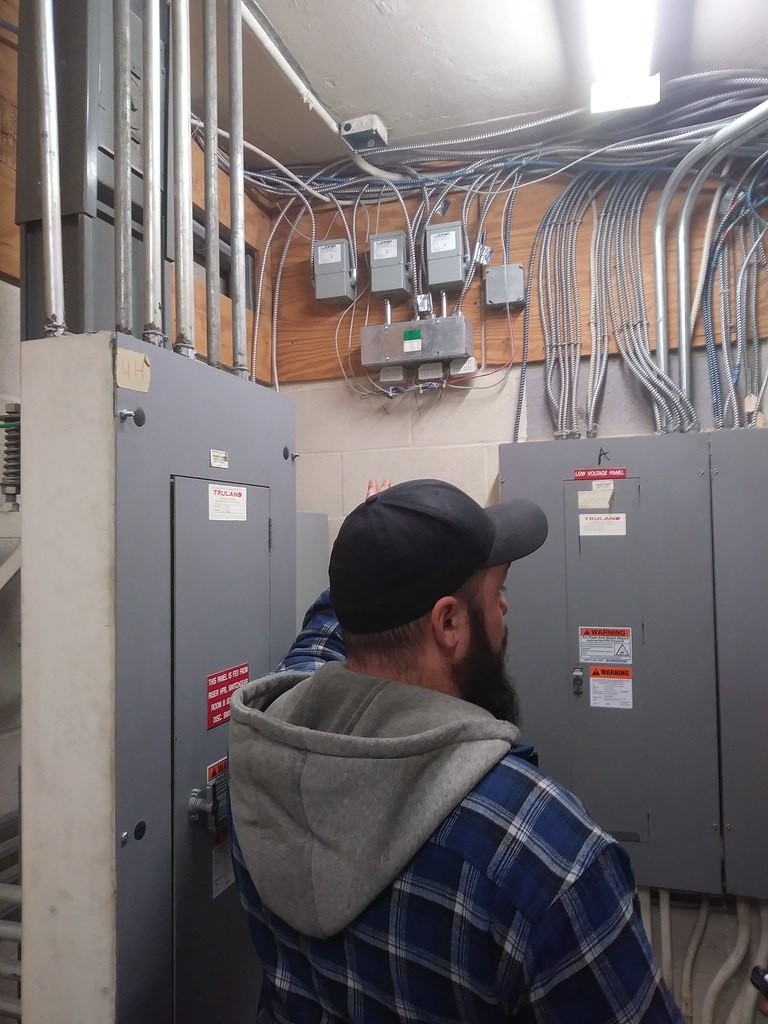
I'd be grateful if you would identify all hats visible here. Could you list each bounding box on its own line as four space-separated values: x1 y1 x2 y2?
328 479 549 634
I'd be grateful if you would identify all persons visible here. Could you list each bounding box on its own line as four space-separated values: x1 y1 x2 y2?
224 479 687 1024
